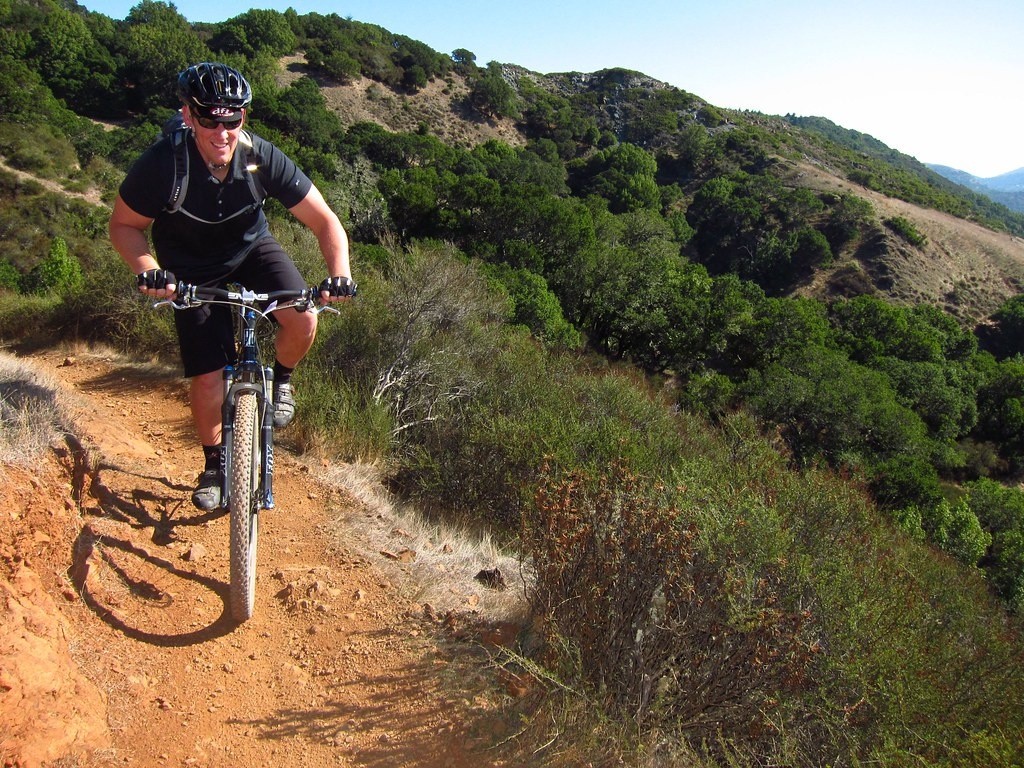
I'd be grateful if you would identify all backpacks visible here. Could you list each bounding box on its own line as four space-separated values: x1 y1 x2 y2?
158 109 272 213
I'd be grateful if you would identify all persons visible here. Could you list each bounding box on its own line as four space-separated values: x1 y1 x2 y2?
108 63 356 511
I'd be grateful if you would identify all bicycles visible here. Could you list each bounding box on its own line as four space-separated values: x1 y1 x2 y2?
153 281 358 622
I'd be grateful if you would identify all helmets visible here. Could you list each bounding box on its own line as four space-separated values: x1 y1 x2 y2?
177 62 252 122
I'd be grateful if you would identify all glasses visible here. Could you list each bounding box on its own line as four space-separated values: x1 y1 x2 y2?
187 106 241 129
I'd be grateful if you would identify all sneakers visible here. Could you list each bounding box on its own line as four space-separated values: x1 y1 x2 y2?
191 468 220 511
273 377 294 429
